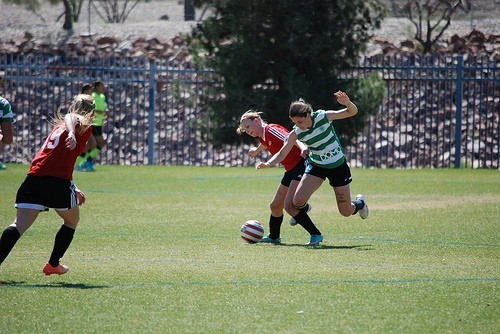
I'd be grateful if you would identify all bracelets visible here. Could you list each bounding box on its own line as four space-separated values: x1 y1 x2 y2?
268 162 271 165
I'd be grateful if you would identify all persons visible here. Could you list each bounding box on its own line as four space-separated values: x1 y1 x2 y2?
236 110 324 246
0 94 96 276
0 82 109 171
254 90 368 225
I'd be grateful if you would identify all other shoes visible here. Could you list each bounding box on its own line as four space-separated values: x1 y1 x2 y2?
74 164 83 171
84 160 95 172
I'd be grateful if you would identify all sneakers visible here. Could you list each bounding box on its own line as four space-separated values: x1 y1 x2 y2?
305 234 323 246
290 205 312 226
42 263 69 275
260 235 281 243
356 195 369 219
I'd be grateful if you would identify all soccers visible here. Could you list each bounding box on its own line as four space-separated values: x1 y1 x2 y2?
240 220 265 245
